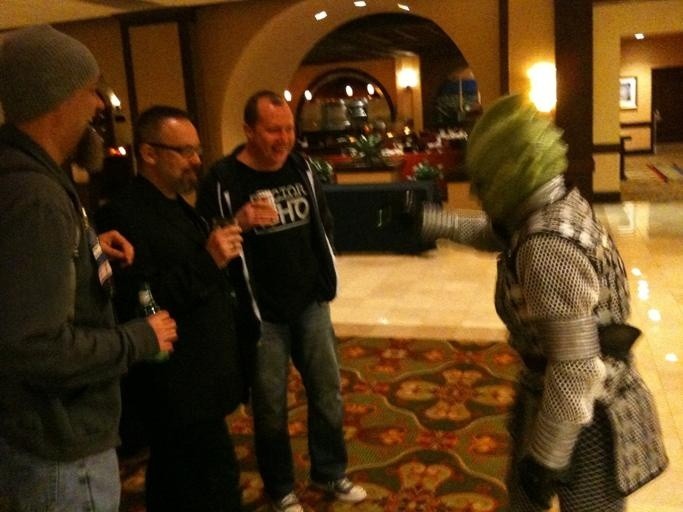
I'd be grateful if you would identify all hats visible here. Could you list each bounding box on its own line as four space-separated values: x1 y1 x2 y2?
0 25 98 123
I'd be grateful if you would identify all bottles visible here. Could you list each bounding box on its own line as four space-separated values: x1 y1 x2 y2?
137 282 171 363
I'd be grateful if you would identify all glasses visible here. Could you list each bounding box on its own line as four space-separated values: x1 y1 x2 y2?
150 143 202 158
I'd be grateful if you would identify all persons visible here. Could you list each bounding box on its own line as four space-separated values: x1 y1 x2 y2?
0 17 180 511
388 94 671 512
96 106 251 511
197 90 369 512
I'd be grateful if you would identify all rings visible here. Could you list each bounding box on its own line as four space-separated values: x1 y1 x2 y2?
229 239 237 252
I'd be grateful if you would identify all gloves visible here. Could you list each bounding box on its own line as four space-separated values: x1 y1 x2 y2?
518 454 570 509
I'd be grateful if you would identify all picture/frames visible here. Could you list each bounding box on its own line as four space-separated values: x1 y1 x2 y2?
616 75 638 113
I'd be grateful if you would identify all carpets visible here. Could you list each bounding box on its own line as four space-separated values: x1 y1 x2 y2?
110 320 558 510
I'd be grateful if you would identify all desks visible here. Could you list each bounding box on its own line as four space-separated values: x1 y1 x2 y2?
292 129 470 202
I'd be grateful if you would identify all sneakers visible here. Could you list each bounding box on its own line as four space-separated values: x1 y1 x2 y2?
314 477 366 501
272 490 303 512
